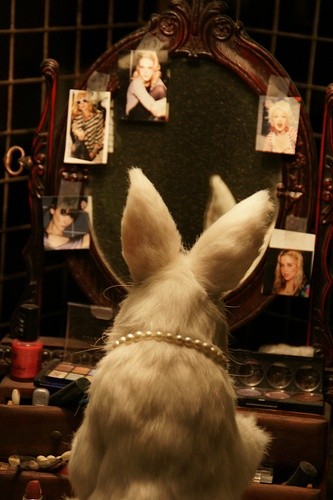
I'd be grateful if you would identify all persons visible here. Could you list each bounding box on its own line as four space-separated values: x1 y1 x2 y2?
71 92 107 162
126 49 169 121
272 248 309 297
263 99 295 152
43 199 89 249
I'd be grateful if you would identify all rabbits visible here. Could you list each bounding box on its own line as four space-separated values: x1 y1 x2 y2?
67 167 275 499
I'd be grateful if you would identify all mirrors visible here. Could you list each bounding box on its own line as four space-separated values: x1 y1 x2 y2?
62 1 318 334
